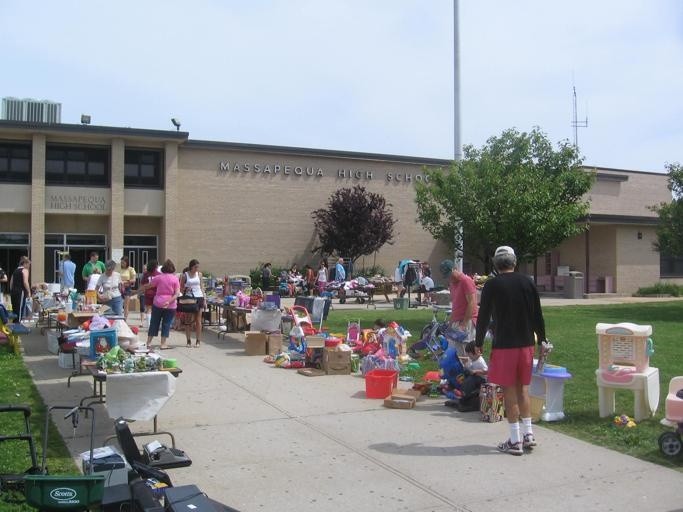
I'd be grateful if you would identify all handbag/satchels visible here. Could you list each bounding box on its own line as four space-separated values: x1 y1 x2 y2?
98 290 112 303
177 295 204 312
144 289 155 299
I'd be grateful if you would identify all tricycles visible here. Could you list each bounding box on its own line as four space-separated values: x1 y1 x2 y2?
278 275 289 298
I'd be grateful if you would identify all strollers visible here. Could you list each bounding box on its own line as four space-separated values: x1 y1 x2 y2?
402 309 452 360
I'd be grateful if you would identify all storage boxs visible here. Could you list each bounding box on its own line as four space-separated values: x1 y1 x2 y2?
47 329 63 354
58 348 77 368
431 293 450 304
304 347 323 369
245 331 266 356
392 297 410 309
365 369 398 398
322 348 351 375
264 332 283 355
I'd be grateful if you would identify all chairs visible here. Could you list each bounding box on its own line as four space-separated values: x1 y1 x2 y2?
114 417 192 468
409 323 444 363
264 293 332 339
0 304 31 357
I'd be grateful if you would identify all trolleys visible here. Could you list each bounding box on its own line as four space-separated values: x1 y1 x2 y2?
288 304 320 369
7 291 32 337
655 375 683 458
319 287 370 305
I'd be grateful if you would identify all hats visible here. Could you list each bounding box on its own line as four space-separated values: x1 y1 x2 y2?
494 246 514 256
440 259 454 279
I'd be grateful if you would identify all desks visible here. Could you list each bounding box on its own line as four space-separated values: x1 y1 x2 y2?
202 292 284 341
33 297 150 388
324 283 390 309
80 356 183 432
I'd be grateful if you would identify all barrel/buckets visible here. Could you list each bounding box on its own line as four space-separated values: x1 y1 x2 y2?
250 301 282 332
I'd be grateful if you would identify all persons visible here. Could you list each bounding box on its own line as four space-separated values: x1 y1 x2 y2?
263 258 345 297
475 246 546 455
59 255 76 292
82 253 207 350
10 256 31 323
440 260 478 359
0 269 8 305
464 340 488 382
395 261 434 301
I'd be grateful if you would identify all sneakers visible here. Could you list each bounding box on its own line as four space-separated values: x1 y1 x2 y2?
521 433 537 447
497 438 523 455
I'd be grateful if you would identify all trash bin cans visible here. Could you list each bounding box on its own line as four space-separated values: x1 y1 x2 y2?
568 271 584 299
